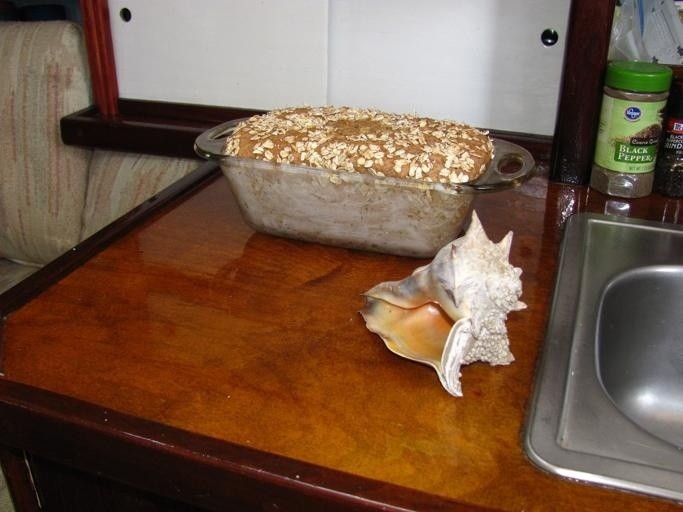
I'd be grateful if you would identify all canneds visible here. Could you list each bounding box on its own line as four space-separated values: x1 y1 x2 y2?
588 61 673 200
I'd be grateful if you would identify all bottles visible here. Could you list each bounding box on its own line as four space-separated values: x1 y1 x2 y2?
589 60 682 200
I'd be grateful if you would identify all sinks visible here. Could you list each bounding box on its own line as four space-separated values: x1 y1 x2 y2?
590 255 683 454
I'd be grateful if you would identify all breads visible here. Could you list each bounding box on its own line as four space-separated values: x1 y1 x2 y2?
222 104 494 183
